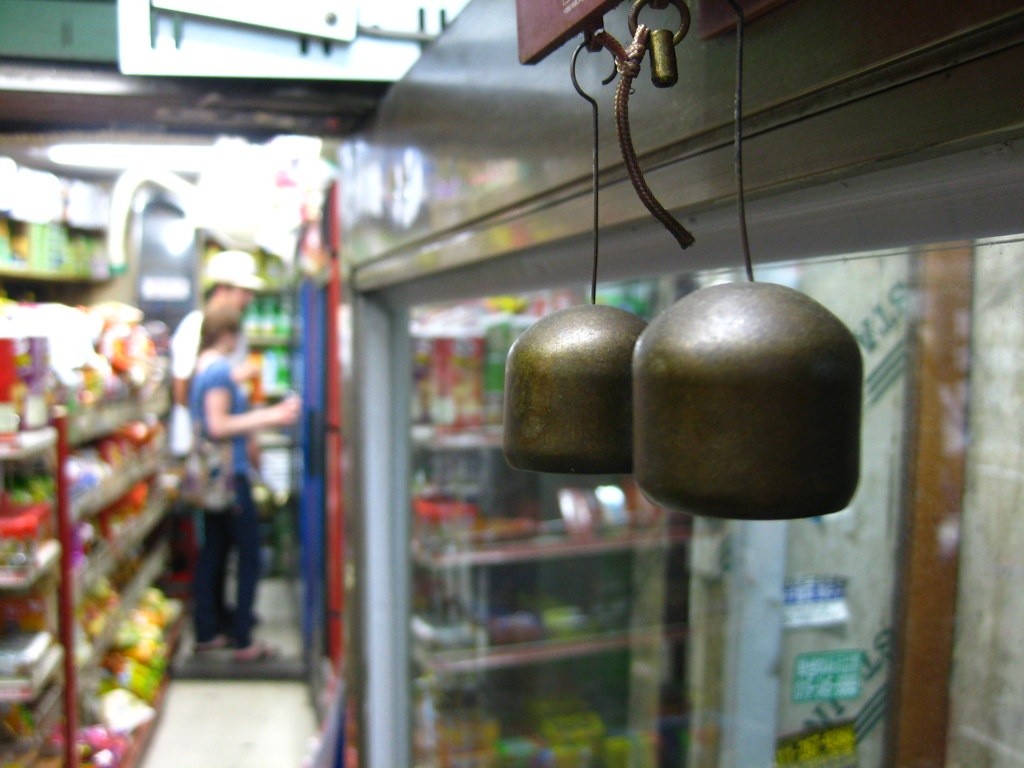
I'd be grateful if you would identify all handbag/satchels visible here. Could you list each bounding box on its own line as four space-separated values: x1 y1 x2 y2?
181 439 237 509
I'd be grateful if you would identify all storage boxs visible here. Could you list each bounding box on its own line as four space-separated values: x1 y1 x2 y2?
0 631 68 728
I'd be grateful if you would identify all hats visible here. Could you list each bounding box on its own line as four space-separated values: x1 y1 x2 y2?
208 251 263 290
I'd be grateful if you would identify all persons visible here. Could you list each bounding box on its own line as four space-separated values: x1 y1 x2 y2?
171 249 266 629
188 310 303 662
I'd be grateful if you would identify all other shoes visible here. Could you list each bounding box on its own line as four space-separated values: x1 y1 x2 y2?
195 634 229 652
236 642 282 662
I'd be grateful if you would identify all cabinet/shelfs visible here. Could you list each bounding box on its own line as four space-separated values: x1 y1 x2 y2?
0 426 65 698
52 383 193 768
